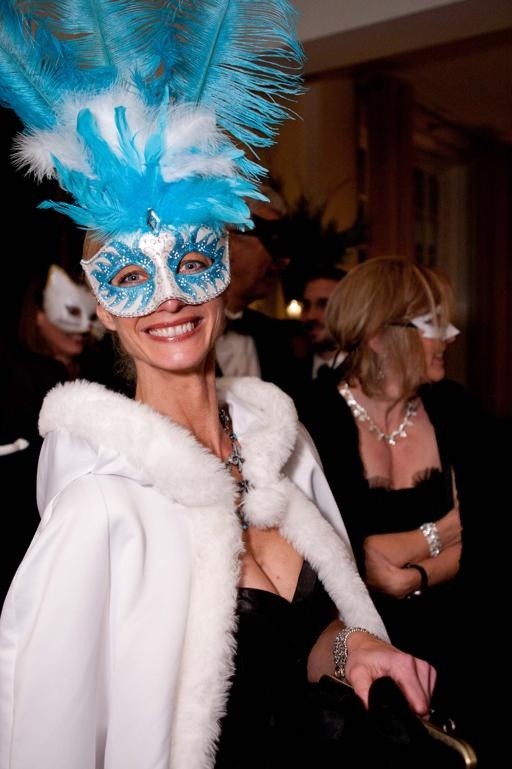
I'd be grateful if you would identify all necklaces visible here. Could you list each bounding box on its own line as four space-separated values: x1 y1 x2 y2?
339 383 418 447
218 409 250 530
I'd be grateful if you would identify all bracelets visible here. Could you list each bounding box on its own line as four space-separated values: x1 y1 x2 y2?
418 522 442 559
401 562 428 600
331 626 376 679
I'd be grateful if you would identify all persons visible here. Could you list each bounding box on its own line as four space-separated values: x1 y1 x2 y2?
1 253 117 572
0 224 479 765
216 263 307 424
294 254 510 767
272 266 352 412
